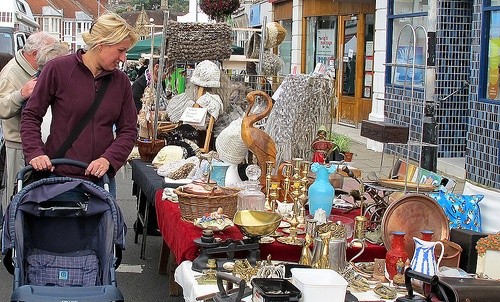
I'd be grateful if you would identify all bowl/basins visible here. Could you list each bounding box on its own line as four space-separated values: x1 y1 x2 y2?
306 215 354 240
137 138 164 163
290 268 348 302
252 277 301 302
234 210 283 237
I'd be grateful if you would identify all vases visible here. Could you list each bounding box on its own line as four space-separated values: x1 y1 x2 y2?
476 250 500 279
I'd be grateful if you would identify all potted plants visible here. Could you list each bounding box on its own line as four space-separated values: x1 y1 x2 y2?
326 131 354 162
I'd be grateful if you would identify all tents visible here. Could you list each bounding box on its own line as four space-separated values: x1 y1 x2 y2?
344 36 357 57
126 35 162 54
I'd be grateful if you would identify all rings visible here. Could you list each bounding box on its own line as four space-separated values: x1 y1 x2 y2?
96 171 99 174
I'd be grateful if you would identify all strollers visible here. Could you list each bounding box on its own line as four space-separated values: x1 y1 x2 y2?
0 158 129 301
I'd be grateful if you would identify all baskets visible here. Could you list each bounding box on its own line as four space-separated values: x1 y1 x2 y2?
174 184 241 226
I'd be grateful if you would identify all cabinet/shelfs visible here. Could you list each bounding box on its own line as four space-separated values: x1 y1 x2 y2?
376 23 440 193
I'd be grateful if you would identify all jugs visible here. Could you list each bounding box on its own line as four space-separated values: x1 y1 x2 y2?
312 239 365 271
411 237 445 277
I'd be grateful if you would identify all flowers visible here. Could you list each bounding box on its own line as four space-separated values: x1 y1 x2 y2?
475 231 500 258
198 0 241 22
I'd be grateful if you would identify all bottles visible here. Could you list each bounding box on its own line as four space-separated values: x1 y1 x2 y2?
308 164 336 218
387 229 435 280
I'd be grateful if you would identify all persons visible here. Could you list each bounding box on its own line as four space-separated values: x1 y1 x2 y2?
199 207 223 223
343 61 356 93
132 46 163 236
310 125 332 163
124 58 150 85
396 258 404 274
404 259 410 272
0 31 57 215
37 42 70 143
19 10 137 197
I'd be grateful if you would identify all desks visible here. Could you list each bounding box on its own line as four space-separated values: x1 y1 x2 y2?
171 259 426 302
153 188 388 296
126 158 165 262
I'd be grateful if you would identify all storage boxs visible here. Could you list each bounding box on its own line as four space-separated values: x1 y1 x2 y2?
451 228 488 275
290 267 349 302
250 277 302 302
360 120 410 144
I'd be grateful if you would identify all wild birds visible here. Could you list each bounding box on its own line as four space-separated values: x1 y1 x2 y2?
239 89 278 183
